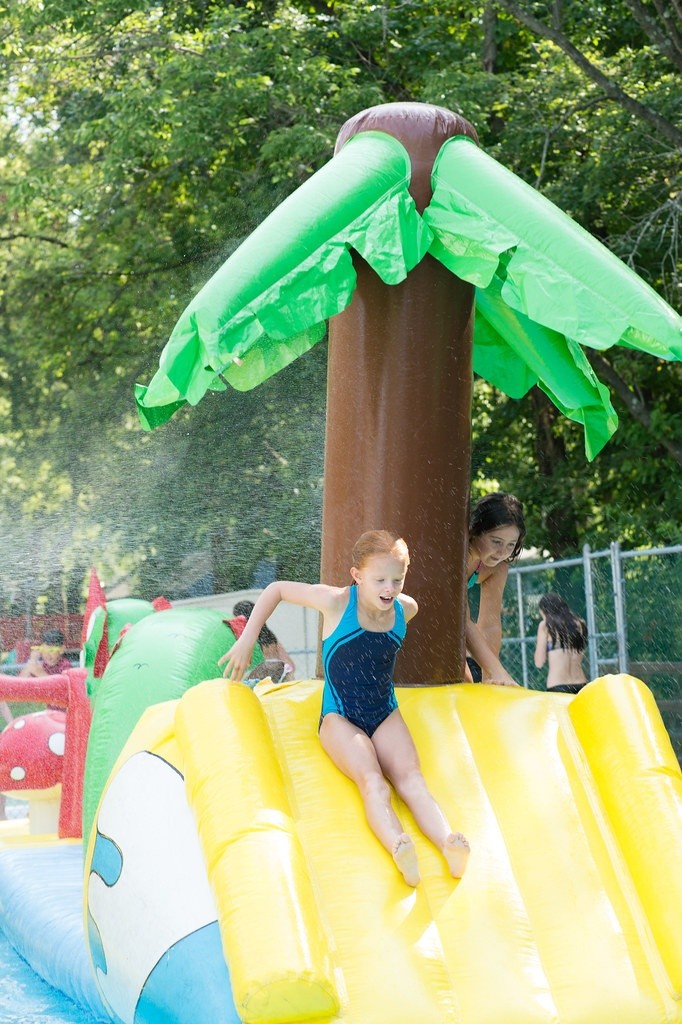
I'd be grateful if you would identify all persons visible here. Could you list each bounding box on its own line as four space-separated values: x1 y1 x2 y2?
534 594 589 694
234 601 294 683
19 631 73 712
466 493 525 687
218 530 470 888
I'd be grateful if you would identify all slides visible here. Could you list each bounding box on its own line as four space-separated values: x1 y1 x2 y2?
87 671 682 1024
